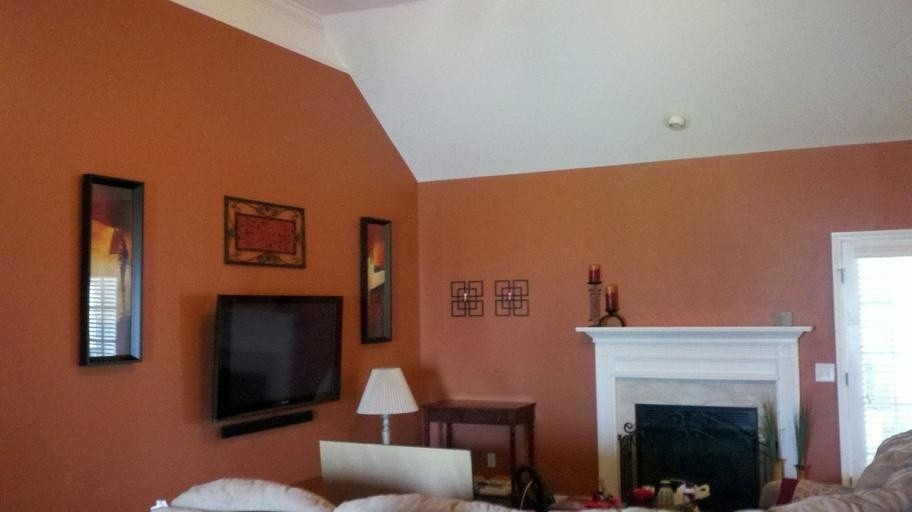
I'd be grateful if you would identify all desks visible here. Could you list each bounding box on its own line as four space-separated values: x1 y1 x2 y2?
417 398 536 505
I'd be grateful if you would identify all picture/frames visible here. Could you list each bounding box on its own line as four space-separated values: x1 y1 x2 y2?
360 216 392 344
79 172 145 367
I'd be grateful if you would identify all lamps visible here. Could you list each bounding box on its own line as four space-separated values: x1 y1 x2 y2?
354 367 419 445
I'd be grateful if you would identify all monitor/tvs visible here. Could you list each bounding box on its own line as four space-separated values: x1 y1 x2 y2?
213 294 344 420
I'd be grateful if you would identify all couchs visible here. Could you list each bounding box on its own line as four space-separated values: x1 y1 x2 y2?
736 429 912 512
154 477 523 511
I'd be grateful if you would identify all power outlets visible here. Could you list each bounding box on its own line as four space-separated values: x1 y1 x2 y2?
815 362 836 384
